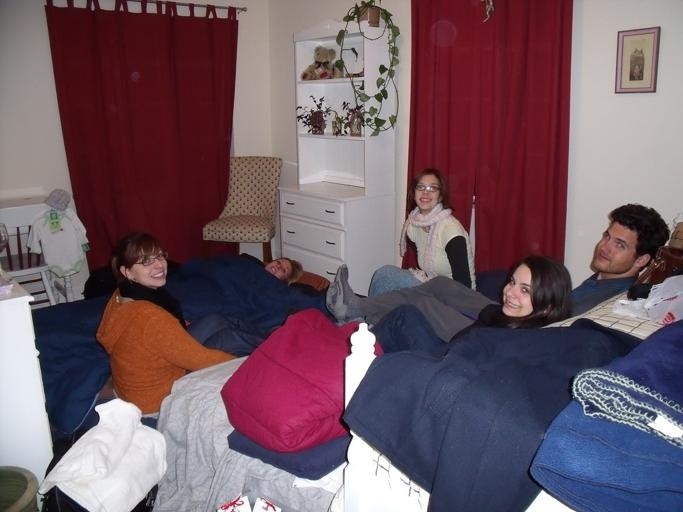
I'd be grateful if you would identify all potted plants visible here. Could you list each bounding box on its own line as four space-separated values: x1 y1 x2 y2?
335 1 402 138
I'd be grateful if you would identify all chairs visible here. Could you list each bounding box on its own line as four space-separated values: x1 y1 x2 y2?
0 225 56 307
203 156 283 263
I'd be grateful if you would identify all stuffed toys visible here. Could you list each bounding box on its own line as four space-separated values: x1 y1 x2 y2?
299 45 337 81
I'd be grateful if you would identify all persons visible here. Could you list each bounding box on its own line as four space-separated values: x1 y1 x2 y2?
368 169 476 297
95 231 237 415
325 204 671 342
264 257 304 287
630 63 643 80
370 255 572 355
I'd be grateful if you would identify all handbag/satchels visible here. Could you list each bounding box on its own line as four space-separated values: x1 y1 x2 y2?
632 246 682 287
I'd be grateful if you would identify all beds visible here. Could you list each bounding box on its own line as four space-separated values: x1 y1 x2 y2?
32 250 372 474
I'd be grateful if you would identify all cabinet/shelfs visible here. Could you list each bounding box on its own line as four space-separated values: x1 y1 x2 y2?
276 27 396 295
1 270 52 511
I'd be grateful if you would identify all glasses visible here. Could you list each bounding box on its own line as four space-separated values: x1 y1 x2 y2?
134 252 169 266
415 185 442 192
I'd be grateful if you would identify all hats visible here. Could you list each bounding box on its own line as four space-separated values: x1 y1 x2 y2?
44 189 71 211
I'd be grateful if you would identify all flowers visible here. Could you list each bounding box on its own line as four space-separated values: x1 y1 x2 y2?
336 101 364 136
296 95 337 135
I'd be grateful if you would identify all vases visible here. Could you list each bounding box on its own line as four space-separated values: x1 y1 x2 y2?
1 466 41 512
311 110 325 134
350 113 362 137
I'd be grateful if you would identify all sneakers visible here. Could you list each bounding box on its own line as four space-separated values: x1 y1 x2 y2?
334 264 353 304
325 282 346 322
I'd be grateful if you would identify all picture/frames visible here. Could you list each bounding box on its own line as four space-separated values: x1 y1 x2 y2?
615 25 661 93
